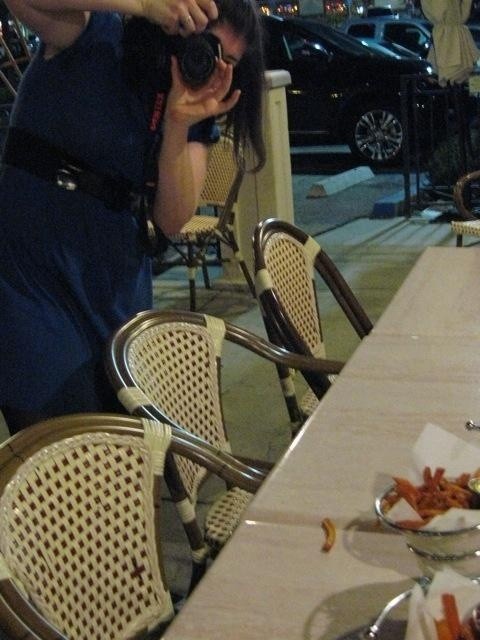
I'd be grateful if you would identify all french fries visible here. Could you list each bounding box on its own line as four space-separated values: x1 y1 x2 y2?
382 466 474 528
322 519 334 550
436 594 473 640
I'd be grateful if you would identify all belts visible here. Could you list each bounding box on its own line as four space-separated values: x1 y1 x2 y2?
1 127 140 214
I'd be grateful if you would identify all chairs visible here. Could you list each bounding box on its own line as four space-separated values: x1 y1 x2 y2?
147 130 258 311
451 170 480 246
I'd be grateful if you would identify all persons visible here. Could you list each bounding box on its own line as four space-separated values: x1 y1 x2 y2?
0 0 270 621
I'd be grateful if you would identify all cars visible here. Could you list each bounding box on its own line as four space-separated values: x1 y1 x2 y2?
256 12 479 172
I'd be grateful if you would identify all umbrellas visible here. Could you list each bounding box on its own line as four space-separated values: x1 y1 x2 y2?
416 0 479 212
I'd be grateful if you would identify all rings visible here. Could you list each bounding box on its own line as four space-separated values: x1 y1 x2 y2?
178 14 192 28
210 84 218 91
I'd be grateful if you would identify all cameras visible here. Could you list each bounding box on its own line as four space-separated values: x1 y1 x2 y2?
164 31 225 85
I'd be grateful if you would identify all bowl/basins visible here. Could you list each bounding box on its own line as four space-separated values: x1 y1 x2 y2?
372 478 480 562
